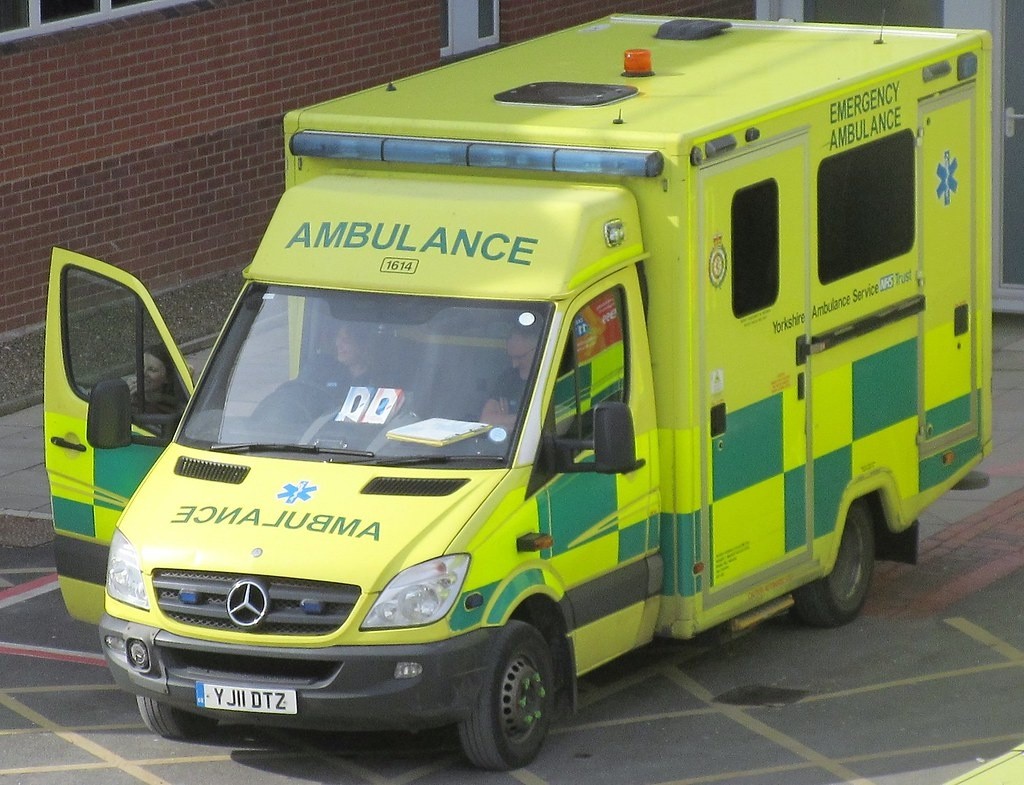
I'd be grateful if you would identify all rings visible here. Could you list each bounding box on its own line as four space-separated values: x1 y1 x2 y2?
501 408 504 411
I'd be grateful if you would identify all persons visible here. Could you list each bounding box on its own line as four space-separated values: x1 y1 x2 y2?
130 344 193 405
299 319 394 400
478 323 587 441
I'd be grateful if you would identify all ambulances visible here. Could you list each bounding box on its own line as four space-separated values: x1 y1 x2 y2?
45 13 993 772
